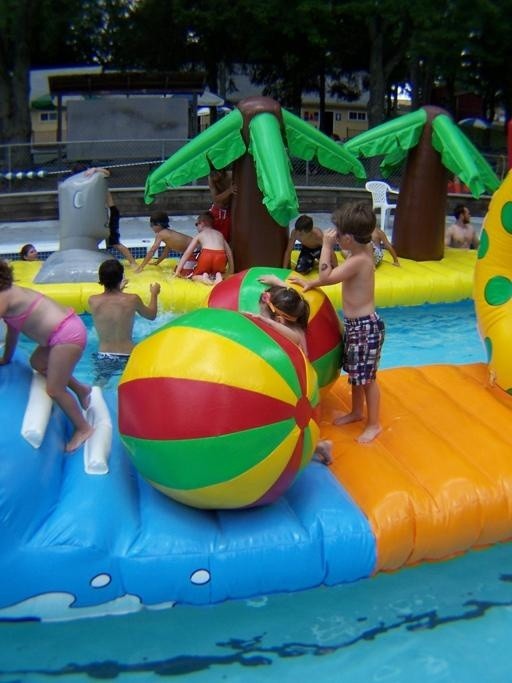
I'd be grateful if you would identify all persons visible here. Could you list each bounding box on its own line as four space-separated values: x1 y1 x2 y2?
287 203 385 443
346 223 400 266
238 272 334 466
20 243 39 260
85 167 138 268
208 161 238 247
134 211 204 278
282 214 339 274
172 211 235 284
86 259 161 387
444 203 480 249
0 259 96 452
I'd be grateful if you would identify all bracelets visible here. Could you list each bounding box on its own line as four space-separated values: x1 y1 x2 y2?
393 258 398 262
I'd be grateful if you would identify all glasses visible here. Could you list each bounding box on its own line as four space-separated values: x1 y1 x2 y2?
194 221 203 226
